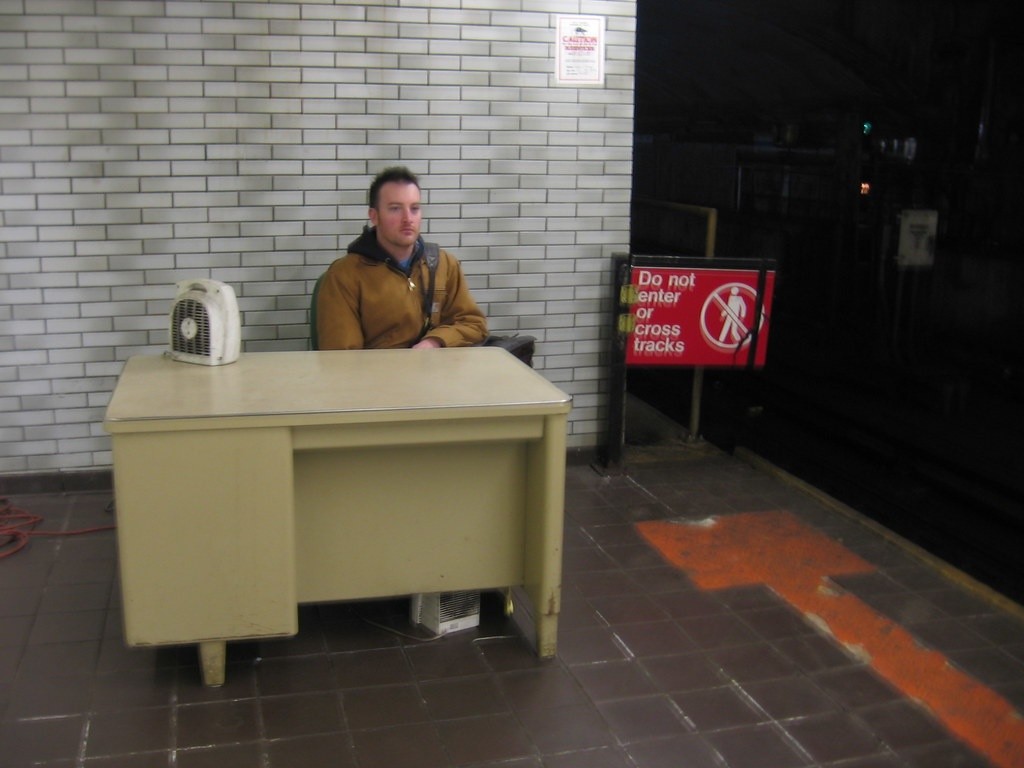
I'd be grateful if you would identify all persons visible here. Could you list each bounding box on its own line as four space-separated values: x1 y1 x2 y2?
312 166 488 351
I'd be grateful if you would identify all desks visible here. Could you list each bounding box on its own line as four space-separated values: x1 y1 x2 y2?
104 346 573 686
